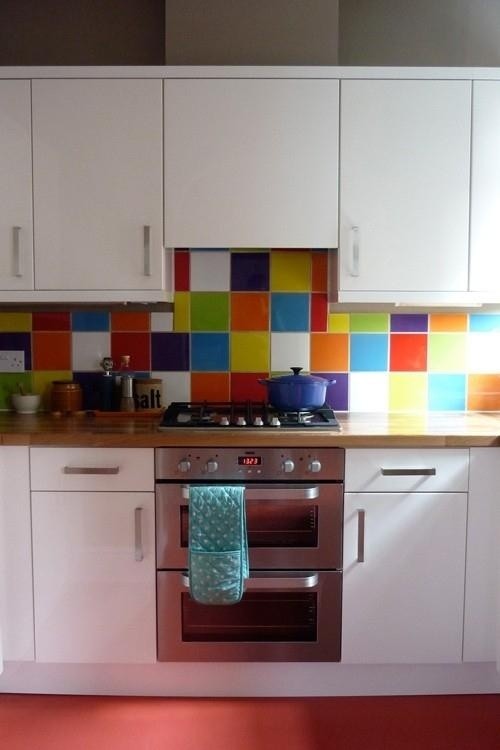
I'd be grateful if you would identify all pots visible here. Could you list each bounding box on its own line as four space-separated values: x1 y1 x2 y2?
257 366 337 412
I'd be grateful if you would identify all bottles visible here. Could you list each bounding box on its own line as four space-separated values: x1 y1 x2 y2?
119 355 134 397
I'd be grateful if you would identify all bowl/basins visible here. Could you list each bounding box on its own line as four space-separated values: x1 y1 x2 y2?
11 393 40 414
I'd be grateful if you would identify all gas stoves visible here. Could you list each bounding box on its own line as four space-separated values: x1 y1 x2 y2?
157 400 342 431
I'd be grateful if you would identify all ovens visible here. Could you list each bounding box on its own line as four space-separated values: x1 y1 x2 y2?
154 446 345 663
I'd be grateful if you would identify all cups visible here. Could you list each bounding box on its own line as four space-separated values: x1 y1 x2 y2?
51 380 83 411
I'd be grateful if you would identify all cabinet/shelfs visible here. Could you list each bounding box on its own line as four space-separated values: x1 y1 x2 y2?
344 448 499 666
2 447 158 666
